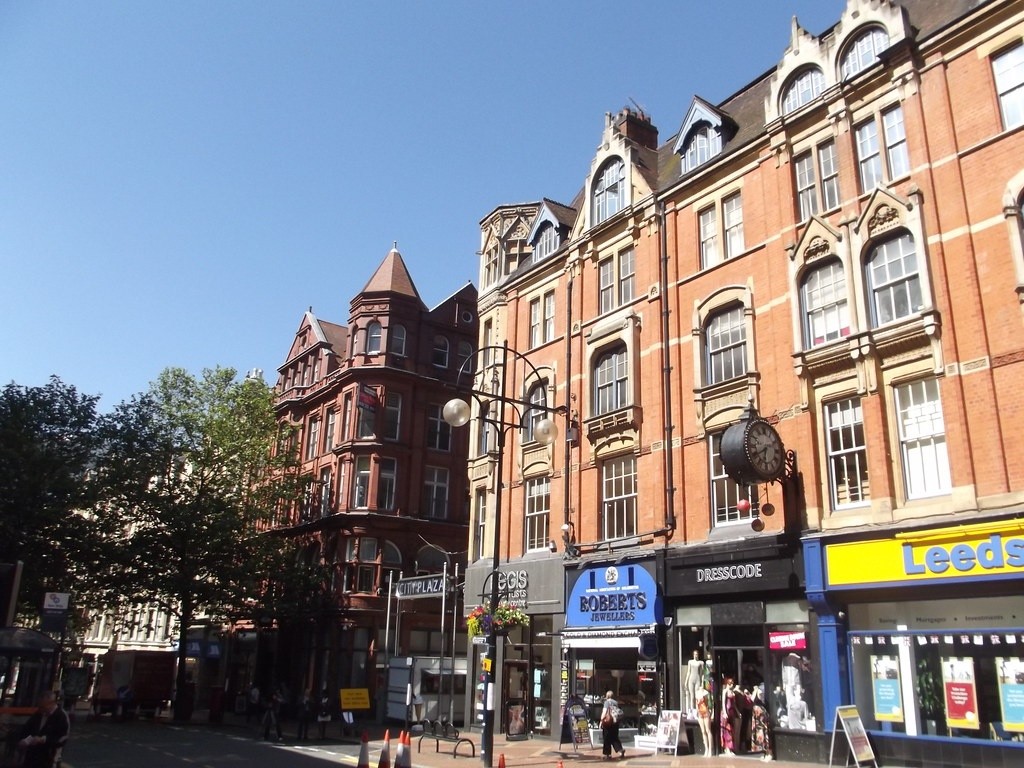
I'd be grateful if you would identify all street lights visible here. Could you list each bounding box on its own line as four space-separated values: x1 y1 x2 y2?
439 341 568 768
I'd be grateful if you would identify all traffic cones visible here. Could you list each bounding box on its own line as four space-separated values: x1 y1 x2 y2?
376 729 391 768
397 732 413 768
356 730 371 768
498 754 507 768
393 729 407 768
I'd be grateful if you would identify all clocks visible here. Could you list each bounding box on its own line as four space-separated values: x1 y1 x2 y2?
746 419 787 485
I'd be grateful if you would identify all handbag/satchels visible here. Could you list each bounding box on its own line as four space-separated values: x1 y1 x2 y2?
601 701 624 727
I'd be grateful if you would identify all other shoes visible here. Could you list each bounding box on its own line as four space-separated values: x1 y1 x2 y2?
620 749 625 758
603 756 612 760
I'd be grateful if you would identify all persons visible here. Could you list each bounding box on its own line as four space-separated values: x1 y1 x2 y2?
295 688 314 739
781 651 802 701
246 688 261 723
599 691 625 759
317 690 331 740
116 683 128 722
16 689 70 768
744 682 772 761
719 679 747 756
685 650 705 709
695 686 713 757
263 692 284 740
